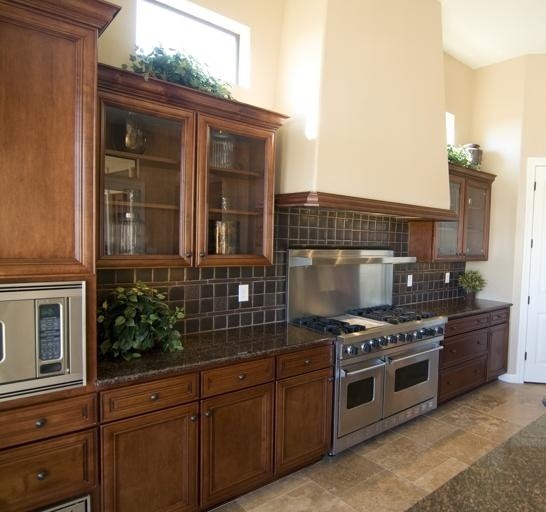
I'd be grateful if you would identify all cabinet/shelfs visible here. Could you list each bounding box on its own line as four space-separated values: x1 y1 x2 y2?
0 393 100 512
96 90 276 269
488 308 509 378
274 344 335 476
99 372 201 512
199 356 276 511
408 174 491 260
438 312 490 400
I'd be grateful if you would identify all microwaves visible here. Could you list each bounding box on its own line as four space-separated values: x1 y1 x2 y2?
0 280 86 397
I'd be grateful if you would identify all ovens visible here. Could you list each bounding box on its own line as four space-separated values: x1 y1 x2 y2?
331 318 448 456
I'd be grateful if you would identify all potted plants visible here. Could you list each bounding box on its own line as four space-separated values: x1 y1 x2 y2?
457 269 488 306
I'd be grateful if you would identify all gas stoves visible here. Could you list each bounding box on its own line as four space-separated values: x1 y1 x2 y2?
288 305 437 335
